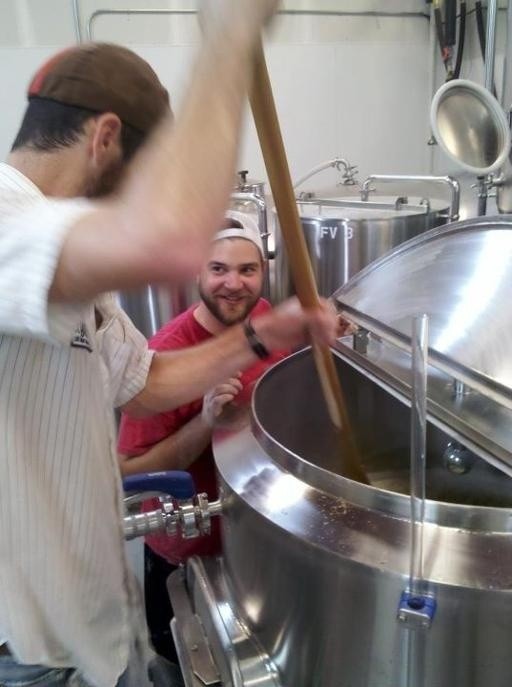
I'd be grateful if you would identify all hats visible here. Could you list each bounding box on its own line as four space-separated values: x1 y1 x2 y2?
28 42 174 134
210 209 265 261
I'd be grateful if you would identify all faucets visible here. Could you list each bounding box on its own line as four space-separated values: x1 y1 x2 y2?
361 175 459 220
292 159 359 190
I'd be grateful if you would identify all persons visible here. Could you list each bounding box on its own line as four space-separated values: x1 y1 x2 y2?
107 209 362 687
1 2 291 686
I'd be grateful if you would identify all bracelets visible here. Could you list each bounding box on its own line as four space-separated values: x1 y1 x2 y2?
240 316 272 363
